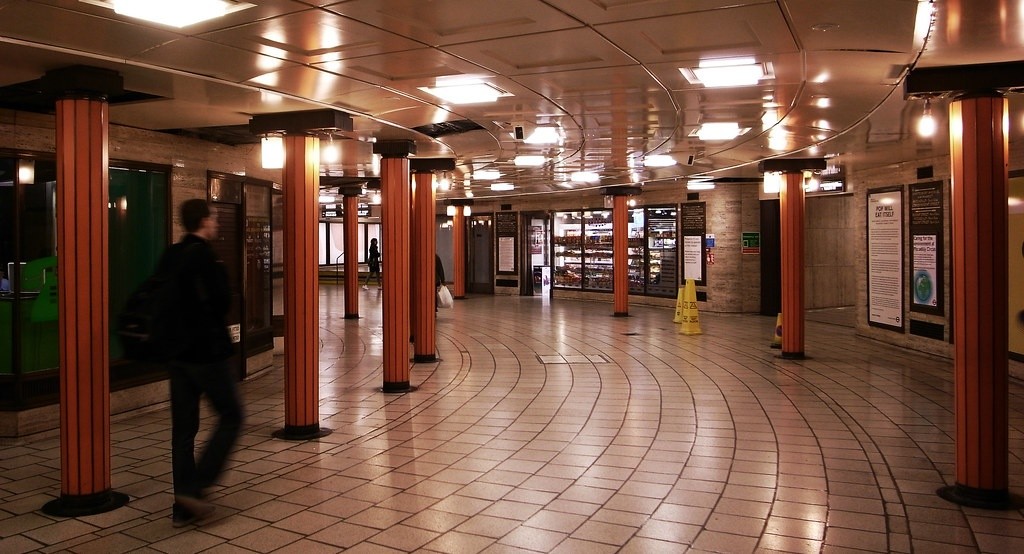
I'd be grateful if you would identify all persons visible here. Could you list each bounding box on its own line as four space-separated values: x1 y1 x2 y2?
362 238 382 290
159 199 247 527
435 254 446 317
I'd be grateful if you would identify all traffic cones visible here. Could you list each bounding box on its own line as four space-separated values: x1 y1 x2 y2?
678 278 703 335
671 287 683 324
770 311 782 348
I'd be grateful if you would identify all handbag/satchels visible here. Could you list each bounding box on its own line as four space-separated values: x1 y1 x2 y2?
368 259 374 267
436 284 453 308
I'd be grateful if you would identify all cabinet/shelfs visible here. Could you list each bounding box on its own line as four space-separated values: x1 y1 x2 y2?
556 221 644 288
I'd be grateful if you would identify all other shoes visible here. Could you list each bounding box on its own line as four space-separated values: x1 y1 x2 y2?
170 496 215 528
362 285 370 290
377 286 382 290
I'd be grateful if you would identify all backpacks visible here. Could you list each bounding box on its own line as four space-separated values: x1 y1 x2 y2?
120 242 200 359
